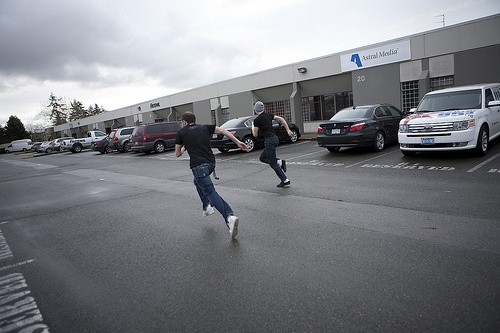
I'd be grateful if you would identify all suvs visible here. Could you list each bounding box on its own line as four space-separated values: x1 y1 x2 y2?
397 83 500 158
130 121 182 155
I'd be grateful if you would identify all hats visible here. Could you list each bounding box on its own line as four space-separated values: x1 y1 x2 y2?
253 101 265 111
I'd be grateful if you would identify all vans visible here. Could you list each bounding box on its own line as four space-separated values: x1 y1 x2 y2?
4 139 34 154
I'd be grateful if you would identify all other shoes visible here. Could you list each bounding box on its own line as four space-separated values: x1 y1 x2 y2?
281 160 287 172
228 215 239 239
277 180 290 187
203 205 215 217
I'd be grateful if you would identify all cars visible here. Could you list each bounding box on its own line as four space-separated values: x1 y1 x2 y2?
31 125 135 155
209 115 301 153
316 102 409 152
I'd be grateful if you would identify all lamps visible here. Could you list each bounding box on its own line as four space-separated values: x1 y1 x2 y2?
298 67 307 73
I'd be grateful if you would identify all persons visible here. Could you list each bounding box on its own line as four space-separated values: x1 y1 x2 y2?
252 102 294 187
175 112 249 238
